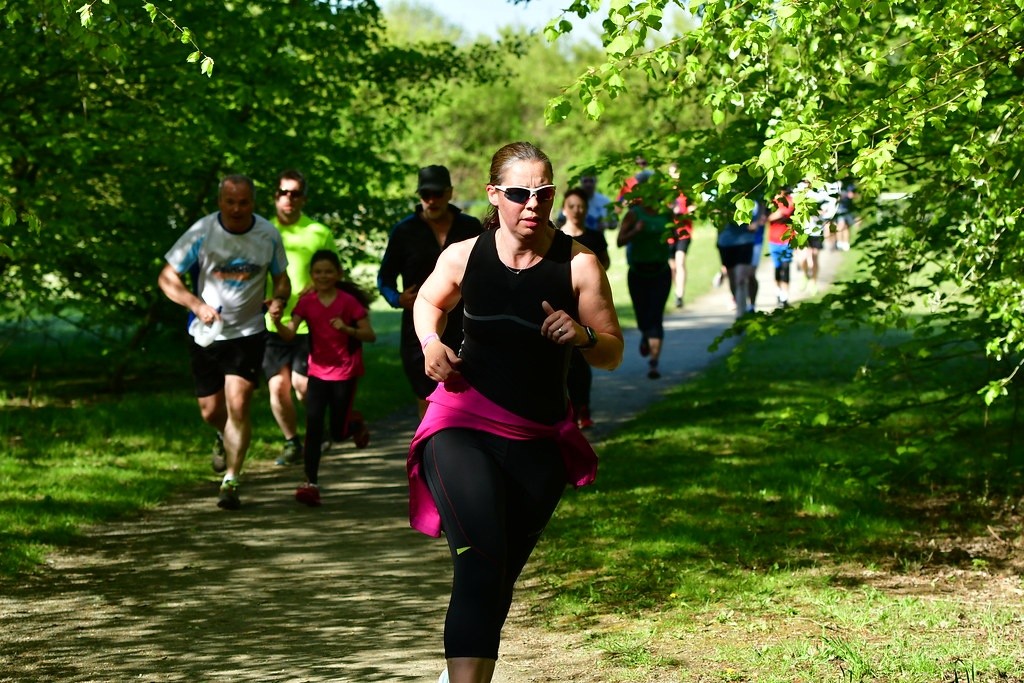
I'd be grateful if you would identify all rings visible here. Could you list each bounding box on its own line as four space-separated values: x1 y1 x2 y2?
205 316 209 319
559 329 563 335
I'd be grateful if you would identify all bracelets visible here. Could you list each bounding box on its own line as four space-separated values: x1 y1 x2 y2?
354 329 356 336
276 296 286 307
421 333 440 351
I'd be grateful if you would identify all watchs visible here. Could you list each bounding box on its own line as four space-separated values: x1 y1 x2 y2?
573 326 598 349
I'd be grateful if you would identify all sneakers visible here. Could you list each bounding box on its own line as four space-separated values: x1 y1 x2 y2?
275 442 305 466
577 416 595 431
217 479 240 511
353 410 369 448
297 486 322 509
212 431 227 475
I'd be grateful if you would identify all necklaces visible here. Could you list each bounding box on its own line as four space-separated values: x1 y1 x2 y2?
504 253 539 274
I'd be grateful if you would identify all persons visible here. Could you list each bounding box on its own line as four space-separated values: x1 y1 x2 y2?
559 188 610 428
377 165 485 420
617 184 677 379
556 174 618 233
666 168 696 307
616 155 647 213
261 172 339 464
799 181 855 279
713 197 767 318
407 142 623 683
768 190 795 304
158 174 291 508
269 249 376 506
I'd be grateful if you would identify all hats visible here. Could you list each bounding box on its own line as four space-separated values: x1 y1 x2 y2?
187 316 223 346
415 164 451 193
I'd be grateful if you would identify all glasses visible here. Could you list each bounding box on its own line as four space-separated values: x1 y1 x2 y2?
494 184 556 204
417 190 447 200
278 189 302 198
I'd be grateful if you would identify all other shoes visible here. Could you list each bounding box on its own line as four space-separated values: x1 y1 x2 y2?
712 271 723 288
675 297 682 307
647 367 661 379
639 337 650 357
